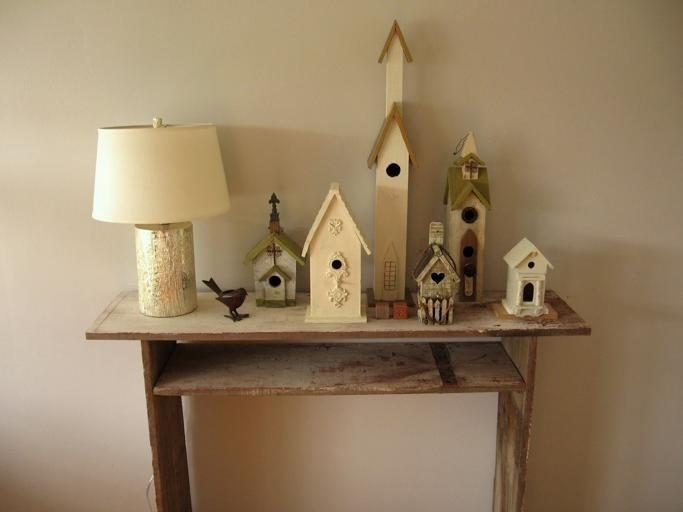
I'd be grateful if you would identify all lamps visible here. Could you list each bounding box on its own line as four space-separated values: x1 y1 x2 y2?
92 119 230 318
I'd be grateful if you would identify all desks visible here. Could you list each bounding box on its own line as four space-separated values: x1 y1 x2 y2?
86 290 591 512
